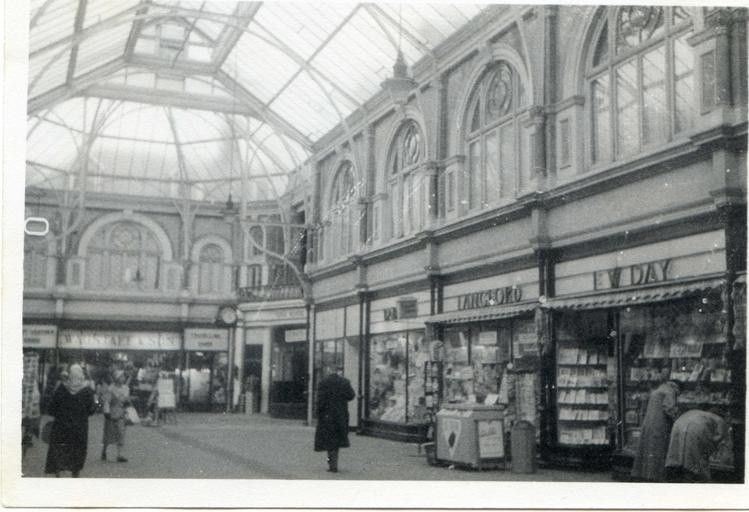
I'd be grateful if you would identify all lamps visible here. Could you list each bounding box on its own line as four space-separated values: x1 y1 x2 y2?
220 0 238 224
379 2 420 105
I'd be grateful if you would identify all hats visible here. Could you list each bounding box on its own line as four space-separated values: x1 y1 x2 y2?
111 369 124 383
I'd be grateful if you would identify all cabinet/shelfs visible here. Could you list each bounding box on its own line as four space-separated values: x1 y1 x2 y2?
549 304 620 462
621 300 733 473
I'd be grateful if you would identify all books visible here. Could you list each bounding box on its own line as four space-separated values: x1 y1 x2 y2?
558 343 612 445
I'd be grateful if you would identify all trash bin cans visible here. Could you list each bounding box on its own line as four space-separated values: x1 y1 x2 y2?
437 405 505 472
511 421 535 475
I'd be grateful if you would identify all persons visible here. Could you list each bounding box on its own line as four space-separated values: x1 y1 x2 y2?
630 378 681 482
664 407 733 482
100 369 130 462
314 361 355 472
35 362 97 477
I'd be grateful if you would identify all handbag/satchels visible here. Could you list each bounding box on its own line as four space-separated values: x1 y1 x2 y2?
123 404 142 426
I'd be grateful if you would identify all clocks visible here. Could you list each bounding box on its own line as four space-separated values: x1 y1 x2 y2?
217 304 239 325
